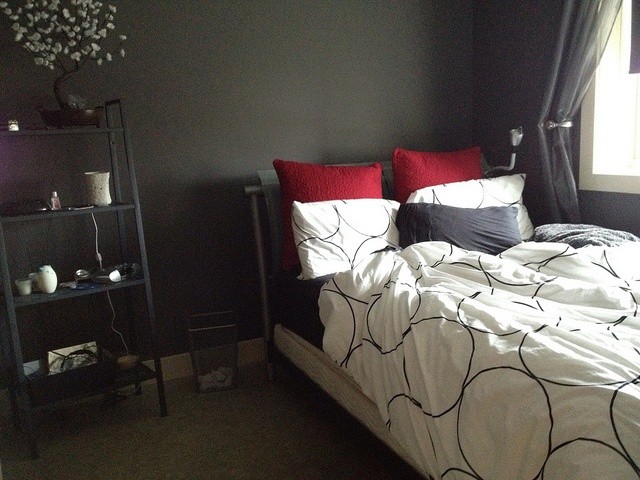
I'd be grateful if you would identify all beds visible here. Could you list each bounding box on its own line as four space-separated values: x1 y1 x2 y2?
245 145 640 480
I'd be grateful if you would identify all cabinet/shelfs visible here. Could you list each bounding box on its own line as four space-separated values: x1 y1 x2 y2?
1 98 169 461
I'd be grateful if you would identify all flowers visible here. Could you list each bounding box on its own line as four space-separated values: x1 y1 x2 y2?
1 0 126 71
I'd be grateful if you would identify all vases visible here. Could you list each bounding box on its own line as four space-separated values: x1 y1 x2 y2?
82 170 113 207
53 69 104 128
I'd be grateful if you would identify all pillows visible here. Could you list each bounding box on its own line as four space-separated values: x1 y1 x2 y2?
390 147 487 195
398 198 525 256
414 172 534 240
272 159 384 260
287 199 403 277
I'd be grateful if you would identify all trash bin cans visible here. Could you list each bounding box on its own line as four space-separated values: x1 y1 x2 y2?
184 310 239 393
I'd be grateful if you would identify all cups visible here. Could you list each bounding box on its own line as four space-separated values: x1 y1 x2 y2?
14 278 32 297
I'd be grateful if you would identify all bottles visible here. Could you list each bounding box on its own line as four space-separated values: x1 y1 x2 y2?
50 191 62 211
